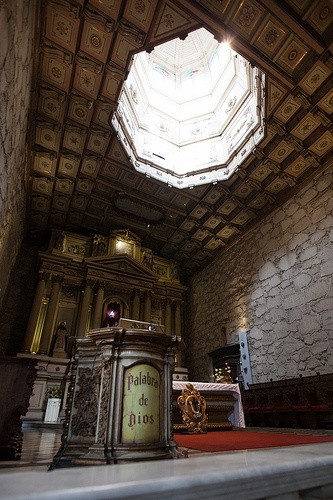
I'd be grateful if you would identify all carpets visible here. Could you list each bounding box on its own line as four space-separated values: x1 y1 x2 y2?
171 427 333 452
